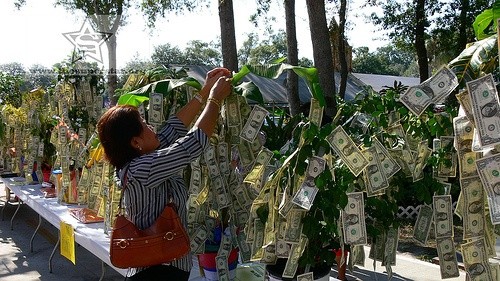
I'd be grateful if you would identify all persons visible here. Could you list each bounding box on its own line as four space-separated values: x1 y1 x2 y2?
97 66 232 280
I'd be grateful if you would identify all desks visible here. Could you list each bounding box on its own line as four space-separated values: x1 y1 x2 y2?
1 175 344 281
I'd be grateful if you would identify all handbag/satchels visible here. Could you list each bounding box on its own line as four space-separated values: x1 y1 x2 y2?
109 165 192 269
